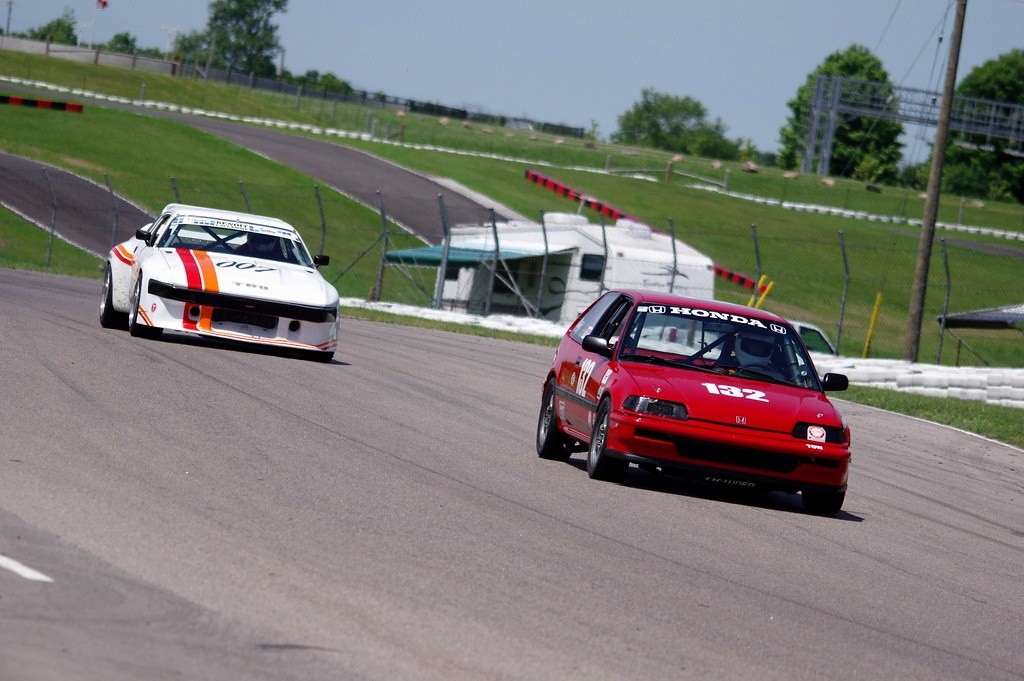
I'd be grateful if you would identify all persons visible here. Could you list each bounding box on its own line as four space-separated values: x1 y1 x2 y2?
712 333 778 377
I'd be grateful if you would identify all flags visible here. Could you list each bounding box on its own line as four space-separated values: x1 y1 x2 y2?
96 0 108 10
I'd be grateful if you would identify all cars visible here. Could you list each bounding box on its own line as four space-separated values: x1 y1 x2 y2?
532 289 854 515
97 202 344 362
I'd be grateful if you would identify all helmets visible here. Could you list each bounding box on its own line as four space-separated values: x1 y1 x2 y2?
736 330 776 372
246 231 277 252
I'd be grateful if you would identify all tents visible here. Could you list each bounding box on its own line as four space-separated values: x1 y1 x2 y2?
383 245 579 270
